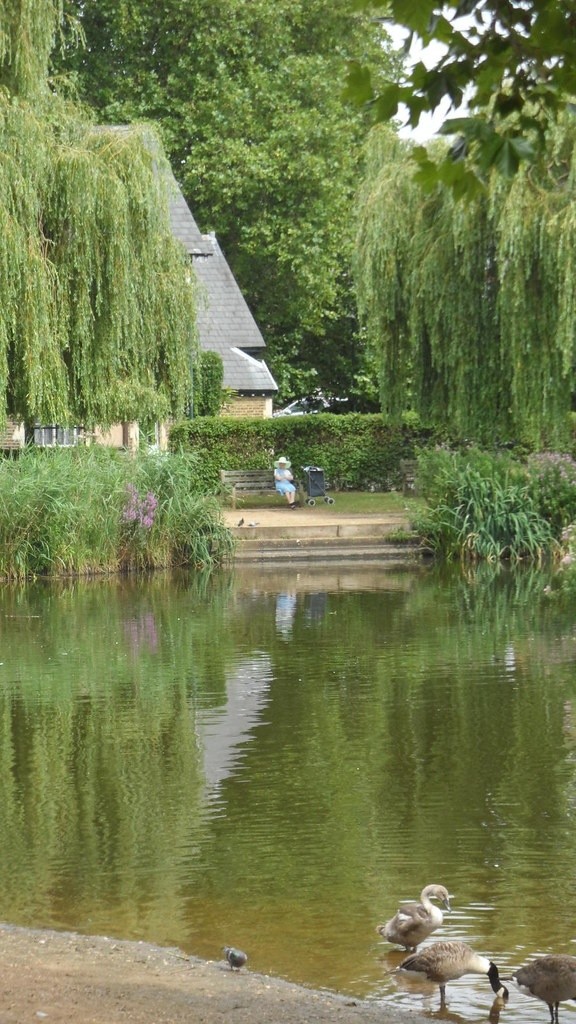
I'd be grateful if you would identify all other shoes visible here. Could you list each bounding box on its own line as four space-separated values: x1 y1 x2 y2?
289 502 296 509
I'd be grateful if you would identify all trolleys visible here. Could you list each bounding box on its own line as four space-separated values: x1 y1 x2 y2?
298 464 335 506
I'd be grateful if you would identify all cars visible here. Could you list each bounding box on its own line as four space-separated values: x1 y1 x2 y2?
275 396 347 420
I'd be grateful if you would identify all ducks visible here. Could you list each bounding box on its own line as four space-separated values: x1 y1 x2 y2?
373 882 453 952
493 952 576 1024
384 940 515 1019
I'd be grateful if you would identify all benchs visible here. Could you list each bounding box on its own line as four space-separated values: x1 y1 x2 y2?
398 459 416 493
218 469 305 509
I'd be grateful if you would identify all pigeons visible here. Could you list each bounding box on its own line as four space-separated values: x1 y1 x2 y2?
219 946 248 973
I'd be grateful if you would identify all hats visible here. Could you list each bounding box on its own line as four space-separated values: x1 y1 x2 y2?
274 457 292 468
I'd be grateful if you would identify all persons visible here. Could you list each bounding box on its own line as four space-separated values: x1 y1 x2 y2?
273 456 297 509
